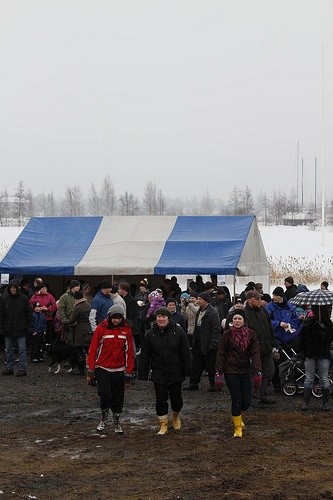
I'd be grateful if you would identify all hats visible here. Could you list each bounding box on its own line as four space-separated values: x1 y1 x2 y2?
198 292 209 303
246 290 264 299
273 287 283 297
181 293 190 300
285 277 293 284
37 283 46 291
231 309 244 319
69 278 171 319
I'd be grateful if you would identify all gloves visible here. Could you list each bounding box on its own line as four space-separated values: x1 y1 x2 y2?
253 374 262 390
215 373 224 390
87 371 97 386
124 374 131 386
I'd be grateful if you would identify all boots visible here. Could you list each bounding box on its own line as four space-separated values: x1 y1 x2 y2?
322 388 329 411
173 411 181 430
301 389 311 410
97 408 109 430
232 415 242 437
158 415 168 434
113 412 123 432
242 421 246 430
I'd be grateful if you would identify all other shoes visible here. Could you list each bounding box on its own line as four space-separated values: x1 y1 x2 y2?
2 368 14 375
253 401 276 408
16 369 26 377
207 387 218 392
183 384 198 390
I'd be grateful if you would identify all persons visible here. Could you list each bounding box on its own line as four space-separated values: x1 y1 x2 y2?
215 309 263 438
226 280 301 401
283 277 333 410
86 304 134 434
134 275 188 380
138 307 193 436
0 279 31 376
87 278 135 386
28 278 56 362
49 280 93 375
177 276 232 391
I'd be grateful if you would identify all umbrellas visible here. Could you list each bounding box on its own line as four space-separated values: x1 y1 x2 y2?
290 289 333 322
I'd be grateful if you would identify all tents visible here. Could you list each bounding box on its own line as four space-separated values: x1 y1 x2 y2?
0 214 270 306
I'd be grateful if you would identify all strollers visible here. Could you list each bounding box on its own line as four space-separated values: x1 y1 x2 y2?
273 335 333 398
